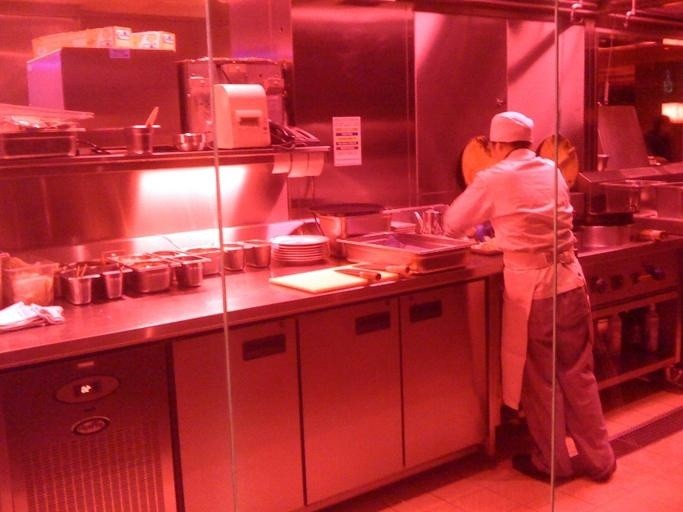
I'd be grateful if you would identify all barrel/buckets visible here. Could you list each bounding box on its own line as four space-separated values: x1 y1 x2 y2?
123 126 153 154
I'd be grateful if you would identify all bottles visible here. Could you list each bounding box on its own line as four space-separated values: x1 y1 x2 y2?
607 314 623 357
643 305 662 352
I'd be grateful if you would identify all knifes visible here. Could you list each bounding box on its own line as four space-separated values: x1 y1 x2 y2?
334 268 382 282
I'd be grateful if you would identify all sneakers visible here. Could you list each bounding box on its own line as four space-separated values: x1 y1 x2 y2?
513 449 616 486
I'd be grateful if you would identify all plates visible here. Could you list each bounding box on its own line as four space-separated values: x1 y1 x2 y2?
271 234 331 263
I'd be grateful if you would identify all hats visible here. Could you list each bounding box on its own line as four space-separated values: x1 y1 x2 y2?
489 111 534 144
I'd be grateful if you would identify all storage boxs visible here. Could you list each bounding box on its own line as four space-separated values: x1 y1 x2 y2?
0 253 60 306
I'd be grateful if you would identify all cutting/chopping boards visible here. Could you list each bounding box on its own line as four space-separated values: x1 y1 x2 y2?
269 263 402 295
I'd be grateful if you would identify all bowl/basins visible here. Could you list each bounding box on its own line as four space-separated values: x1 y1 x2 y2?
173 131 207 152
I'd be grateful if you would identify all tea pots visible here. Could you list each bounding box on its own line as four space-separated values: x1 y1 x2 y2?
413 207 445 236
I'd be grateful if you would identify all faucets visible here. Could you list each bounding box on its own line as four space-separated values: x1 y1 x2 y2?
421 210 440 235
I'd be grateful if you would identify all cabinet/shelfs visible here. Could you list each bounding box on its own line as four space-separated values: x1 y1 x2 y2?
296 275 488 511
169 315 305 511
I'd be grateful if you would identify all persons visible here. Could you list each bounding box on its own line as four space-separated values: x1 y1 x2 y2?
442 112 616 484
